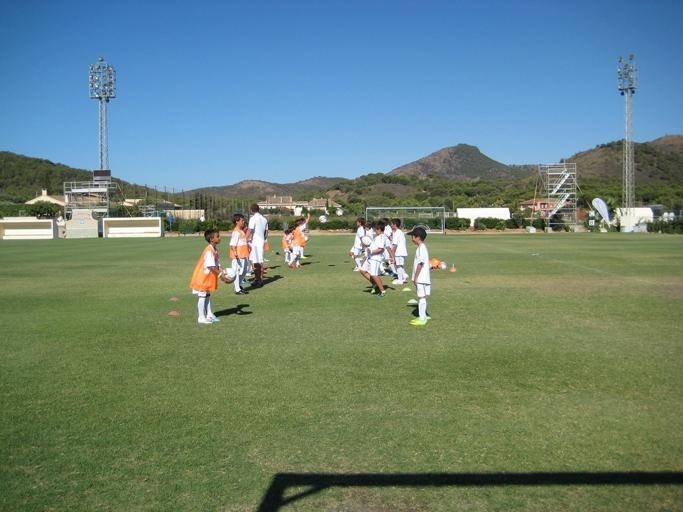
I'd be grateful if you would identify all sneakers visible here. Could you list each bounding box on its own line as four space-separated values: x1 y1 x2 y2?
248 273 264 289
408 317 426 326
368 272 409 297
234 289 249 295
197 315 220 324
284 254 308 271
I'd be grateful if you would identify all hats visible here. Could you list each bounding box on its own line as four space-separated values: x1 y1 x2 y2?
405 227 427 236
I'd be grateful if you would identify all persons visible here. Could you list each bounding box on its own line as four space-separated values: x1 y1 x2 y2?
190 203 431 326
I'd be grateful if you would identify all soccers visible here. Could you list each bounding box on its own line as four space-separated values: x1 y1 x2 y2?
319 216 327 223
441 262 448 269
222 269 237 284
